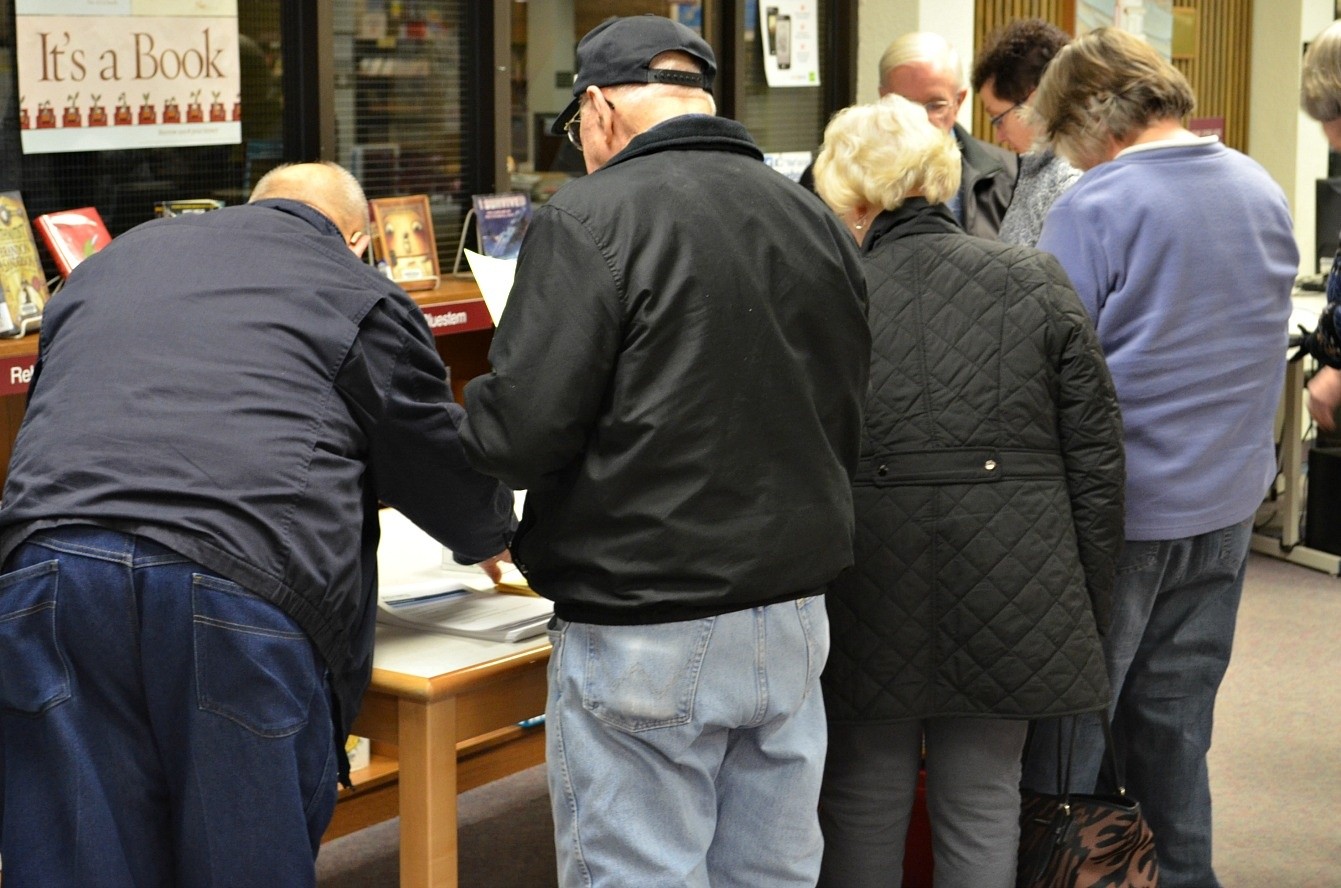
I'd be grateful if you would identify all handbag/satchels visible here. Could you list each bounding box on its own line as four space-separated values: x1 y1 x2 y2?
1024 707 1160 888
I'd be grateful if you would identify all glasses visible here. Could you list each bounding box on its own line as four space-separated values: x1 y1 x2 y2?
986 98 1026 134
924 94 956 121
564 96 615 151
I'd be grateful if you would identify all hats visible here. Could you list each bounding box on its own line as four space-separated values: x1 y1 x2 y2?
552 12 719 134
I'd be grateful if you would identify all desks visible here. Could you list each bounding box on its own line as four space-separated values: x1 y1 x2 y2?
0 271 496 500
319 487 555 888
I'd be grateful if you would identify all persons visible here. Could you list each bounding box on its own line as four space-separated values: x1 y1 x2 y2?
796 11 1341 888
462 18 874 888
0 159 519 886
